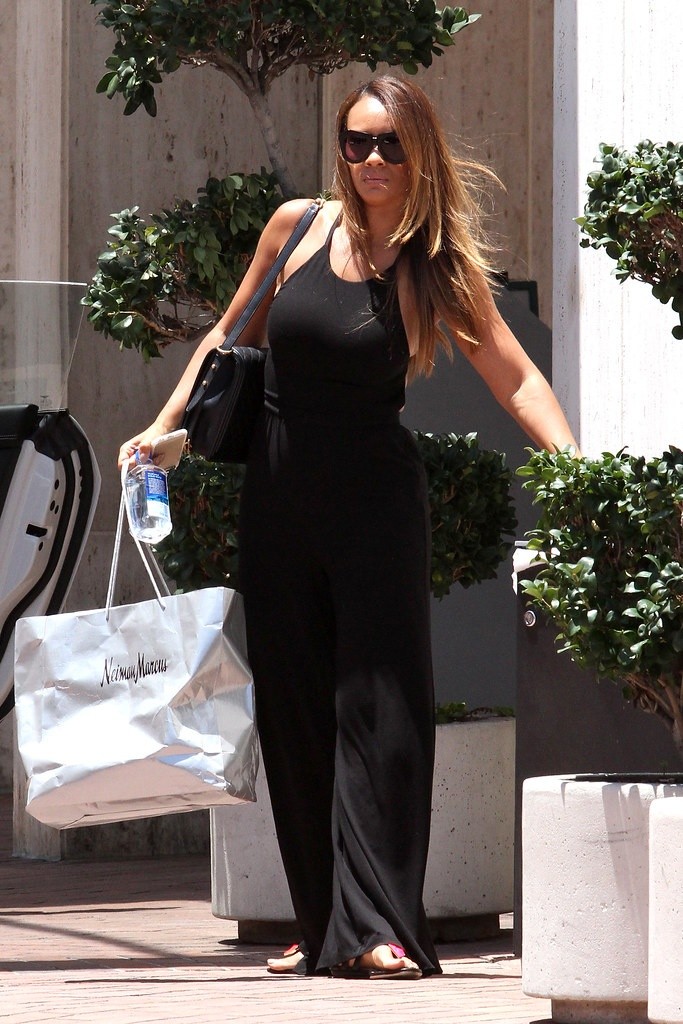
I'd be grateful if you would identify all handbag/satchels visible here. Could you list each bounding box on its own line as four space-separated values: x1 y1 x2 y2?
175 346 269 464
13 459 259 829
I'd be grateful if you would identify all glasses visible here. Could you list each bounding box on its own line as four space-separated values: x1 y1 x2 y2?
338 127 407 164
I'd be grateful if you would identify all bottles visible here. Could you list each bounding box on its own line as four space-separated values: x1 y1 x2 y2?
123 451 173 545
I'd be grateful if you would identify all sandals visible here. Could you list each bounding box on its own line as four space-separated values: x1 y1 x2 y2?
329 944 423 979
267 943 331 975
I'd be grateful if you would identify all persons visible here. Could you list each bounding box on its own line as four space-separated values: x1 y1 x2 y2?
119 76 584 978
